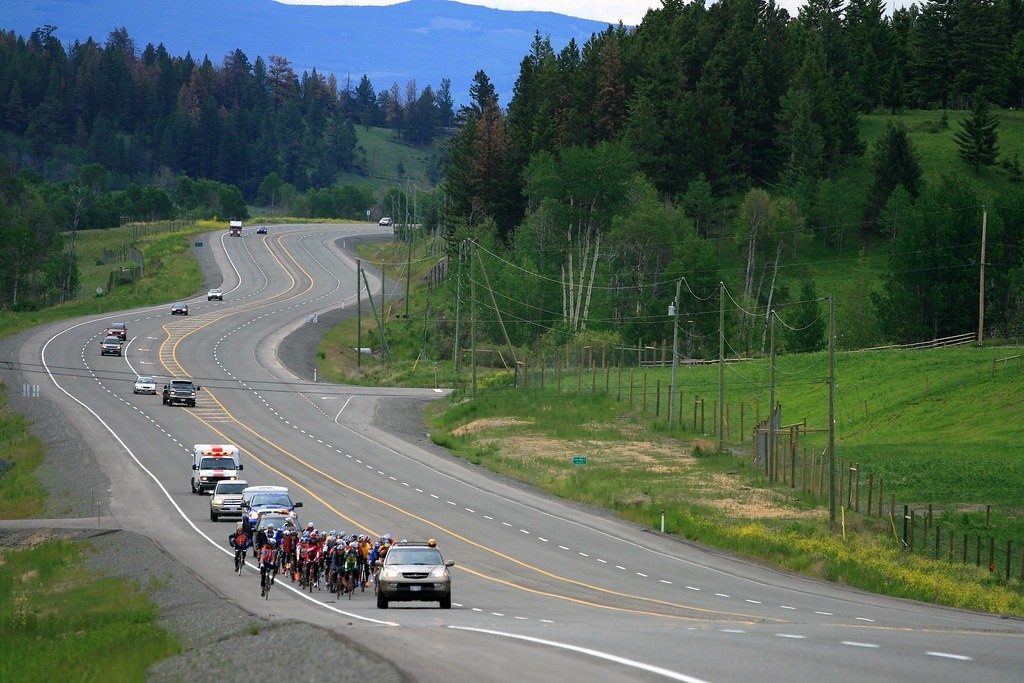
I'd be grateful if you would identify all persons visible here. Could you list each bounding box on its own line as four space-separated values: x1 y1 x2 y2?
228 526 249 573
255 517 396 597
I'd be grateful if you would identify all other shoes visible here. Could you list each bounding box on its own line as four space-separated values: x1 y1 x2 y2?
342 580 349 586
314 583 317 588
295 573 302 581
242 561 245 565
281 568 286 574
365 582 370 587
323 582 329 591
370 576 376 583
235 567 238 572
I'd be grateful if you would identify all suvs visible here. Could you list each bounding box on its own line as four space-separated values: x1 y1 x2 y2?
375 538 455 609
208 479 250 521
250 511 306 557
163 379 200 407
99 335 124 357
107 322 127 341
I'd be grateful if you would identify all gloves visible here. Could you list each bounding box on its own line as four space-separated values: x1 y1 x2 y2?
270 564 274 569
260 565 265 571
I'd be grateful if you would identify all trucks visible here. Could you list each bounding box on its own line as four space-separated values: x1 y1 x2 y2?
190 443 244 496
241 484 303 534
229 220 243 237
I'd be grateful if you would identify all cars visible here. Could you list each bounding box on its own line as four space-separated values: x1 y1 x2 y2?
257 226 267 234
171 301 189 316
379 217 393 227
133 377 158 395
208 289 223 302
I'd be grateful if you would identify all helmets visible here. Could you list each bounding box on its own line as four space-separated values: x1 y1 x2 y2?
268 523 274 528
283 530 299 537
237 526 243 531
286 518 294 523
299 530 393 551
259 526 266 530
268 538 277 544
308 522 315 528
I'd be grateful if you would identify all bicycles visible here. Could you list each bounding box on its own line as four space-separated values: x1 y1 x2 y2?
231 530 380 601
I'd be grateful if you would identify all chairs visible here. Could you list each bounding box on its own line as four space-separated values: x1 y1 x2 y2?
276 498 289 505
